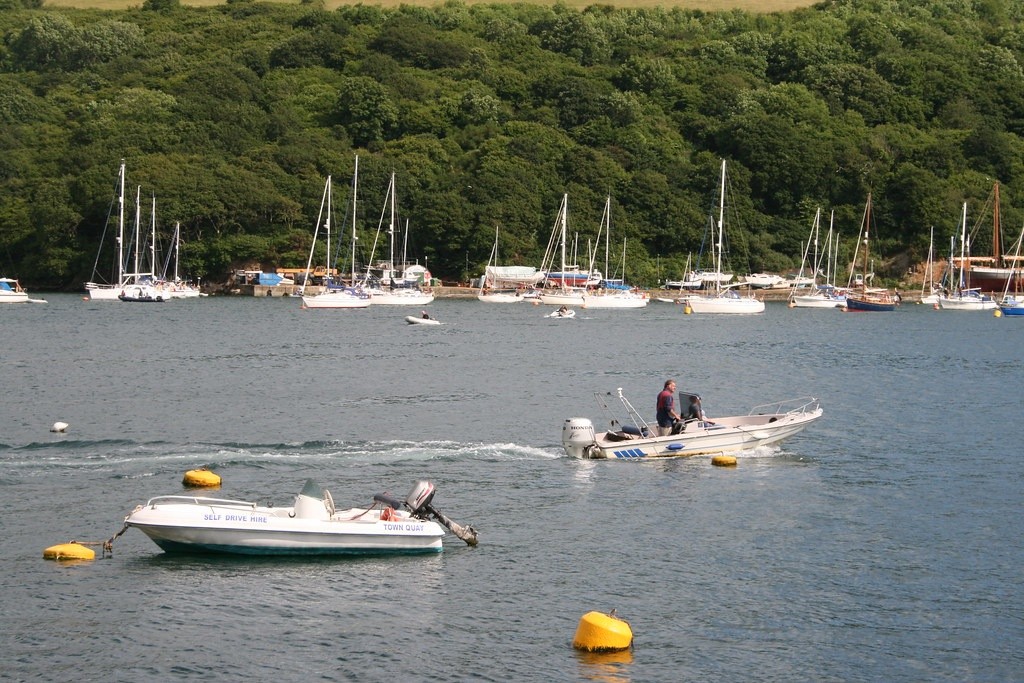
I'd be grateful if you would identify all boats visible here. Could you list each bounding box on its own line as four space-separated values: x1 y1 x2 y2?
551 308 576 319
405 315 441 325
561 386 824 460
124 494 445 558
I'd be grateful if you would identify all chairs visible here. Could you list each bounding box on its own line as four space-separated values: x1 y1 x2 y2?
354 494 400 521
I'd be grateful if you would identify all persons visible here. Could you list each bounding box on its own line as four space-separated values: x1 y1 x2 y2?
656 380 680 436
558 305 567 316
421 310 430 320
689 394 719 427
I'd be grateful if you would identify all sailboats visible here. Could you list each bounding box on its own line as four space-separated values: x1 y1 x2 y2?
665 156 896 314
83 156 201 301
476 193 650 309
920 179 1024 315
297 154 436 308
0 235 49 303
